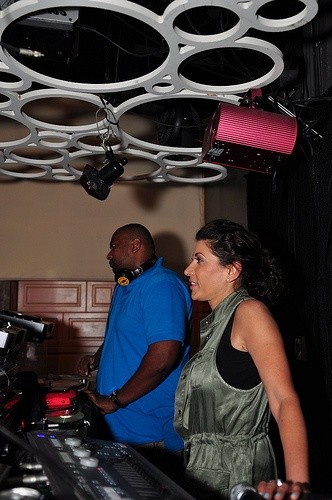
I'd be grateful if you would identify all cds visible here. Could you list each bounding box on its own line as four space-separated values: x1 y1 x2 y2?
47 409 84 423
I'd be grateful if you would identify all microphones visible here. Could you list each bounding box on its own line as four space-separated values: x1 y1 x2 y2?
230 482 266 500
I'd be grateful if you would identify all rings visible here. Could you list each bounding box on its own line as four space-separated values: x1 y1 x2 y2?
276 478 283 488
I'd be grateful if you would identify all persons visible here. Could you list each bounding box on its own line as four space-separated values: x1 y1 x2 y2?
78 223 192 476
174 219 311 500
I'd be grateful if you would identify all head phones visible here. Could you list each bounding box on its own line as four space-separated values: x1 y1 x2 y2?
114 256 158 286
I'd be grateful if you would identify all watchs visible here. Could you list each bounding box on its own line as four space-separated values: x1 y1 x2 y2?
110 389 128 408
